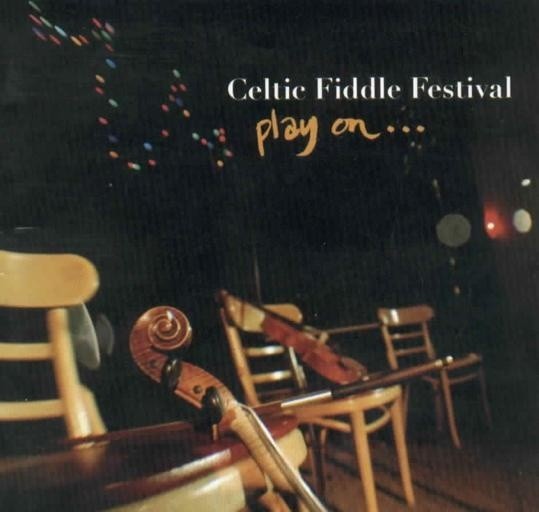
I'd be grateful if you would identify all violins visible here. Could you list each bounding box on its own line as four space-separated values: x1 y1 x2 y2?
230 292 367 388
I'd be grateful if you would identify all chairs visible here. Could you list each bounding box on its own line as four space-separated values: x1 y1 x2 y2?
0 244 309 510
371 303 496 450
212 298 420 511
